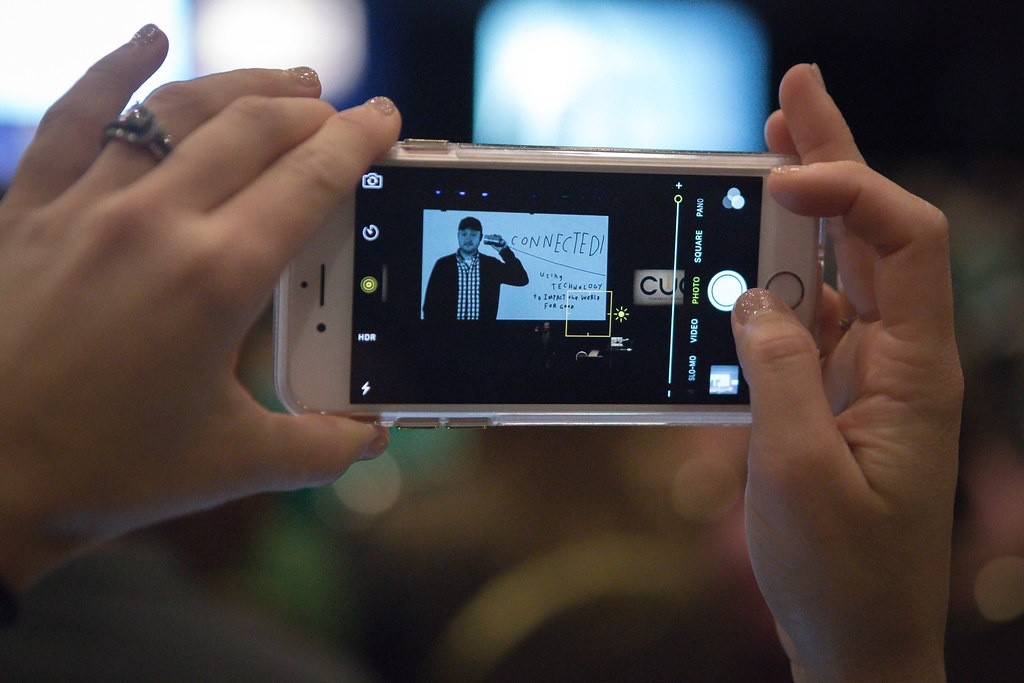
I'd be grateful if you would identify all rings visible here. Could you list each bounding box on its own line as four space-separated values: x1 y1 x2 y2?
103 102 170 161
839 318 855 333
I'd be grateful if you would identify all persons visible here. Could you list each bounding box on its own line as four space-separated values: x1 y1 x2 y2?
423 218 531 320
1 19 964 683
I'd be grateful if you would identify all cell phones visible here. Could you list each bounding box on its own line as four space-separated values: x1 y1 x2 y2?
275 142 823 430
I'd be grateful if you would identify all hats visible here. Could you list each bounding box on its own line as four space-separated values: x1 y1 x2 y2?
457 216 483 231
